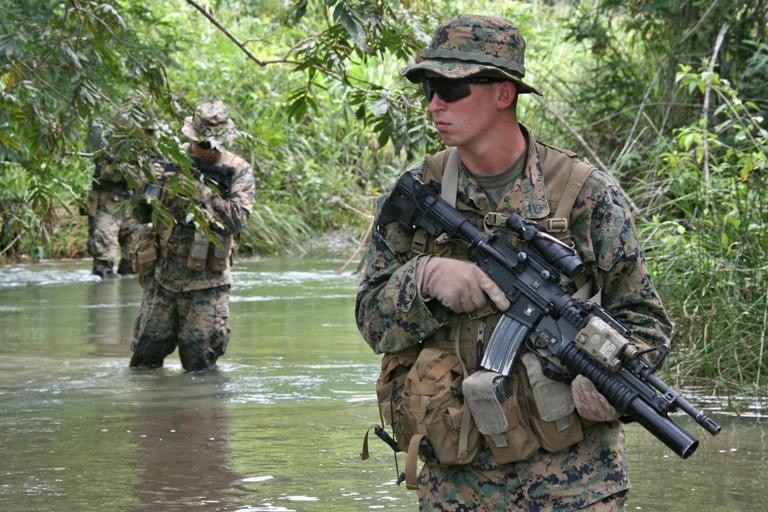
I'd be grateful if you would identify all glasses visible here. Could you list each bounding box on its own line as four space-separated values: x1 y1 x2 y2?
196 142 219 152
422 77 507 102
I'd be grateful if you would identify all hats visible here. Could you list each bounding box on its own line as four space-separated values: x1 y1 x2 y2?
404 14 544 97
181 102 234 148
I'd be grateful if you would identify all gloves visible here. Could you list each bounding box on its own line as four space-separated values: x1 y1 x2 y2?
416 254 510 316
134 163 164 192
179 174 211 201
571 374 622 422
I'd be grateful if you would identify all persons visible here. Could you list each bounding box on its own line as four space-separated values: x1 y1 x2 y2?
126 99 256 376
87 121 151 278
352 12 675 512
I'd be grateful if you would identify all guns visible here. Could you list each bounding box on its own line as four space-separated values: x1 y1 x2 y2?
107 150 234 198
375 172 721 458
